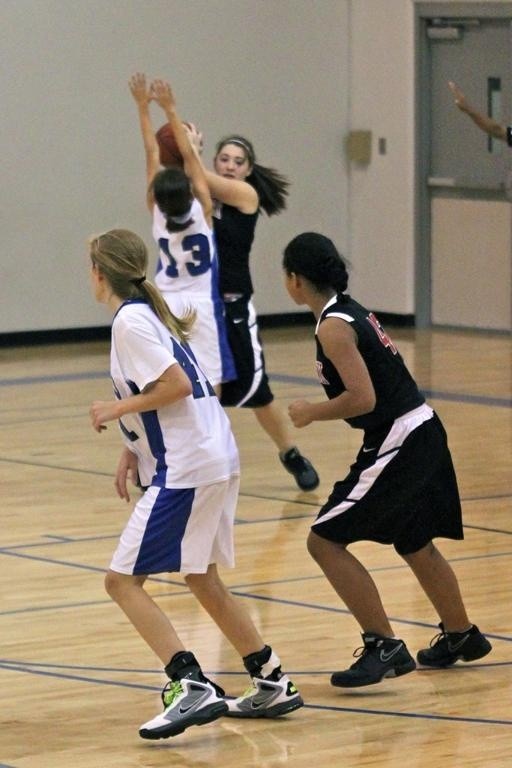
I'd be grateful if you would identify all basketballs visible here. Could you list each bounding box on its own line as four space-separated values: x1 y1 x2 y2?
154 121 203 167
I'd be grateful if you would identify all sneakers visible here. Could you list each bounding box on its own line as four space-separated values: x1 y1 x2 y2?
278 446 319 491
330 631 416 687
417 621 491 666
225 673 304 718
139 677 229 740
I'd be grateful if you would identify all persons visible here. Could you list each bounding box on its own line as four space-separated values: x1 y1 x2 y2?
440 77 510 177
181 116 321 493
124 66 238 415
280 230 496 690
83 225 307 742
